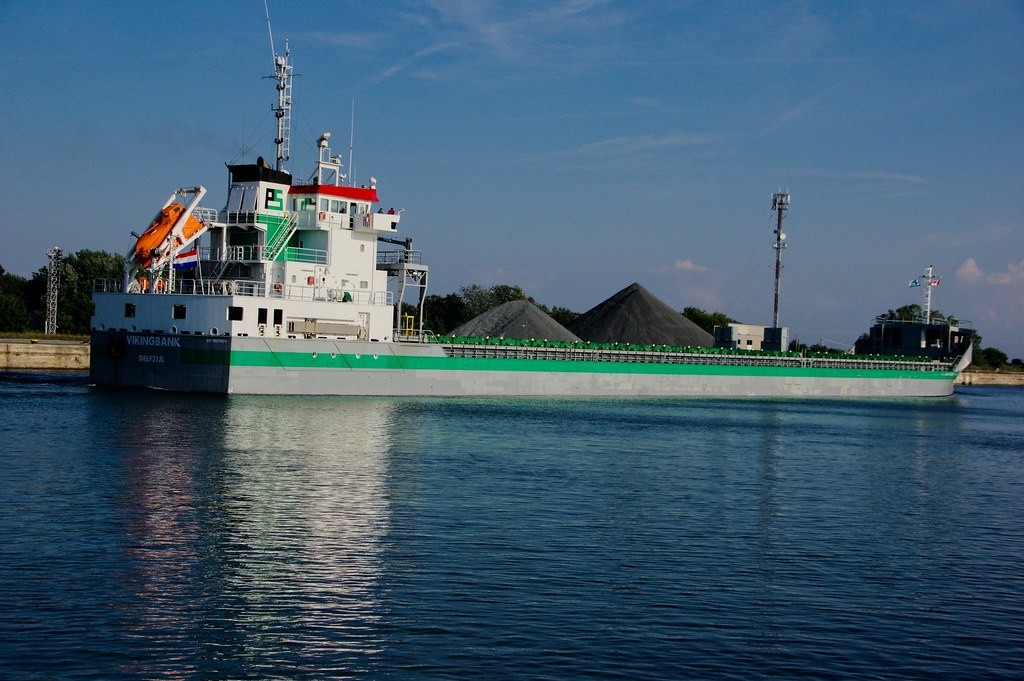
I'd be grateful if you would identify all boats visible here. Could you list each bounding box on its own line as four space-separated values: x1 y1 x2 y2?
90 0 960 397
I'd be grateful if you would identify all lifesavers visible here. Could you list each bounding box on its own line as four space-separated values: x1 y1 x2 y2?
319 211 327 222
274 281 283 293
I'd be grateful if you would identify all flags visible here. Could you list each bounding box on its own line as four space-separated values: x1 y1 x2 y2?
909 279 920 288
928 279 939 286
